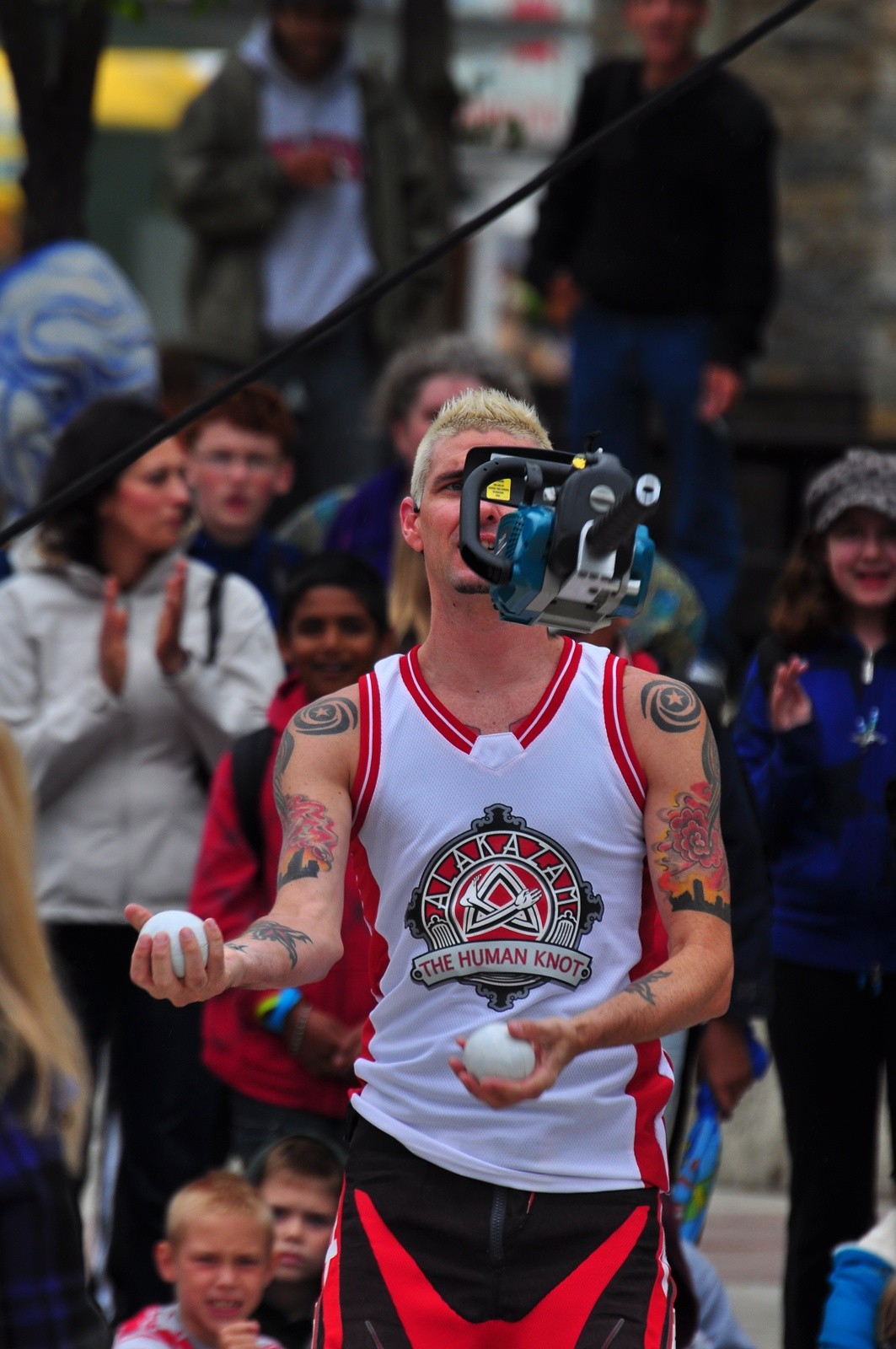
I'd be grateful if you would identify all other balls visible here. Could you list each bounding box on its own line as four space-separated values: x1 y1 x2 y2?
460 1024 537 1093
136 910 213 982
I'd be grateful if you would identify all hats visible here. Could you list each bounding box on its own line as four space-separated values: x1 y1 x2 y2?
803 448 896 541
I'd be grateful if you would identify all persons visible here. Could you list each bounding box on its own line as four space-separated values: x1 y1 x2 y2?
0 727 110 1349
734 448 896 1349
500 0 779 663
112 1134 342 1349
1 339 529 1323
157 0 459 506
125 390 732 1349
